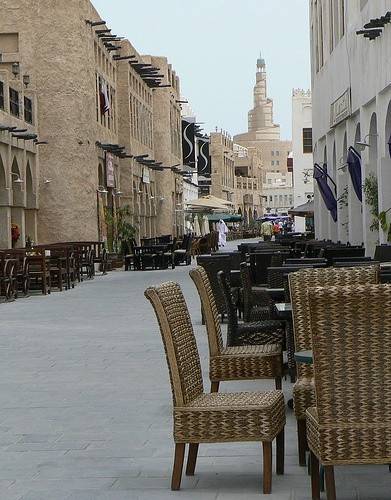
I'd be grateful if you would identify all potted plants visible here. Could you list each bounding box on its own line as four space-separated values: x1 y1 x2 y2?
104 205 140 268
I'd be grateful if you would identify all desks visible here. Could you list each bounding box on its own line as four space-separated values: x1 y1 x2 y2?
274 302 296 383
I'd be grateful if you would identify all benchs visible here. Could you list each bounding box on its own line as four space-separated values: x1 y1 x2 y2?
0 241 108 302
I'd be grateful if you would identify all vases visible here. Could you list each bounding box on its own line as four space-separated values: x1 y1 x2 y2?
13 237 17 248
31 241 34 246
26 242 31 247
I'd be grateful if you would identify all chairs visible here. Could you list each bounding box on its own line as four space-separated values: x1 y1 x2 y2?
122 229 391 500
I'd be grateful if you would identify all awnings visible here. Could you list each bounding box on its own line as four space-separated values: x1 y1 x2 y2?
208 213 240 222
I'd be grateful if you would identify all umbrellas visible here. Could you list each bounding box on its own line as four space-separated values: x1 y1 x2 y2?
181 194 234 210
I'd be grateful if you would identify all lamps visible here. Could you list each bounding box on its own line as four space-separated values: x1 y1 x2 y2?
10 172 24 183
337 157 348 172
23 71 30 89
302 175 313 184
33 177 50 183
356 134 378 151
97 186 181 206
12 60 18 78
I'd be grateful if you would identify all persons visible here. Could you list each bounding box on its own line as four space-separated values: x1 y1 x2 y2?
216 219 228 247
272 220 283 236
261 217 273 241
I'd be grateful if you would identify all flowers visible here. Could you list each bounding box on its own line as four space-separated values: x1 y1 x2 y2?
11 218 22 242
25 233 33 242
